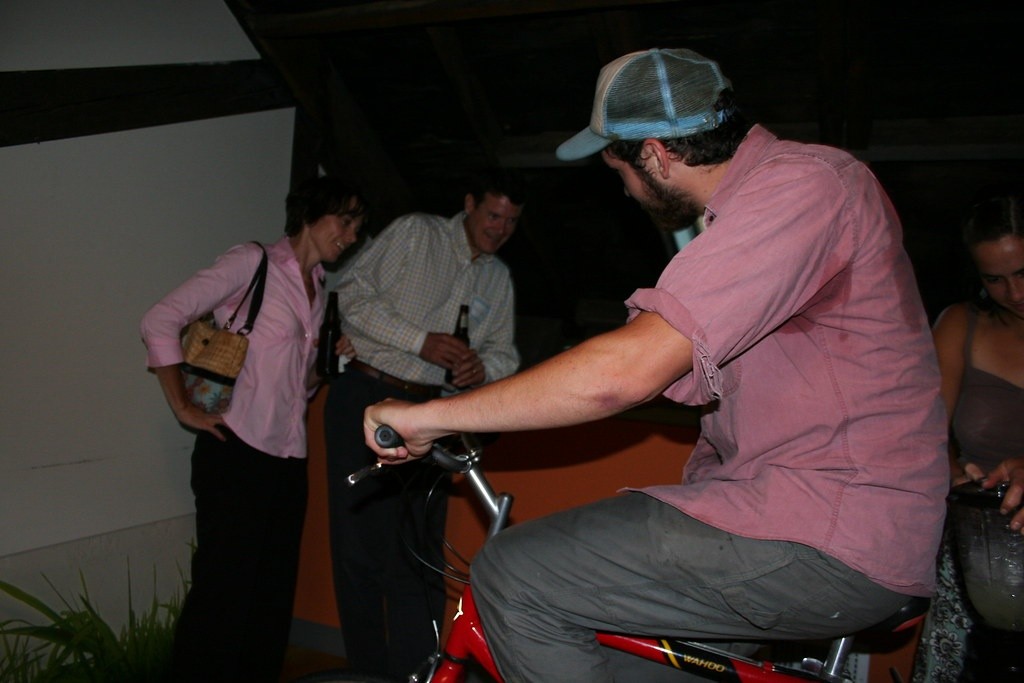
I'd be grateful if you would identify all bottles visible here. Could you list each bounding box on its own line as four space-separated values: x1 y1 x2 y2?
316 292 341 377
446 304 470 383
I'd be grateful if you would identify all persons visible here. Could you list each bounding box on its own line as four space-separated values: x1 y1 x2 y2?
139 174 366 683
364 49 949 683
323 171 521 675
911 199 1024 683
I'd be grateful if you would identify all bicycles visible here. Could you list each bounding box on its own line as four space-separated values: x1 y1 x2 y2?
345 426 937 683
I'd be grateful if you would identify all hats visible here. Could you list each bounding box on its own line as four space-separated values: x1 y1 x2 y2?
556 49 736 161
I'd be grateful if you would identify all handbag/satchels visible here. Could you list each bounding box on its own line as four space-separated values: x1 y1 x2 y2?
179 240 268 413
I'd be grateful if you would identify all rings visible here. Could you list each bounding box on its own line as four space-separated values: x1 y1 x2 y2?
472 368 476 374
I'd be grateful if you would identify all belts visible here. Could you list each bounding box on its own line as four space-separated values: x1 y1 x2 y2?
353 360 430 396
